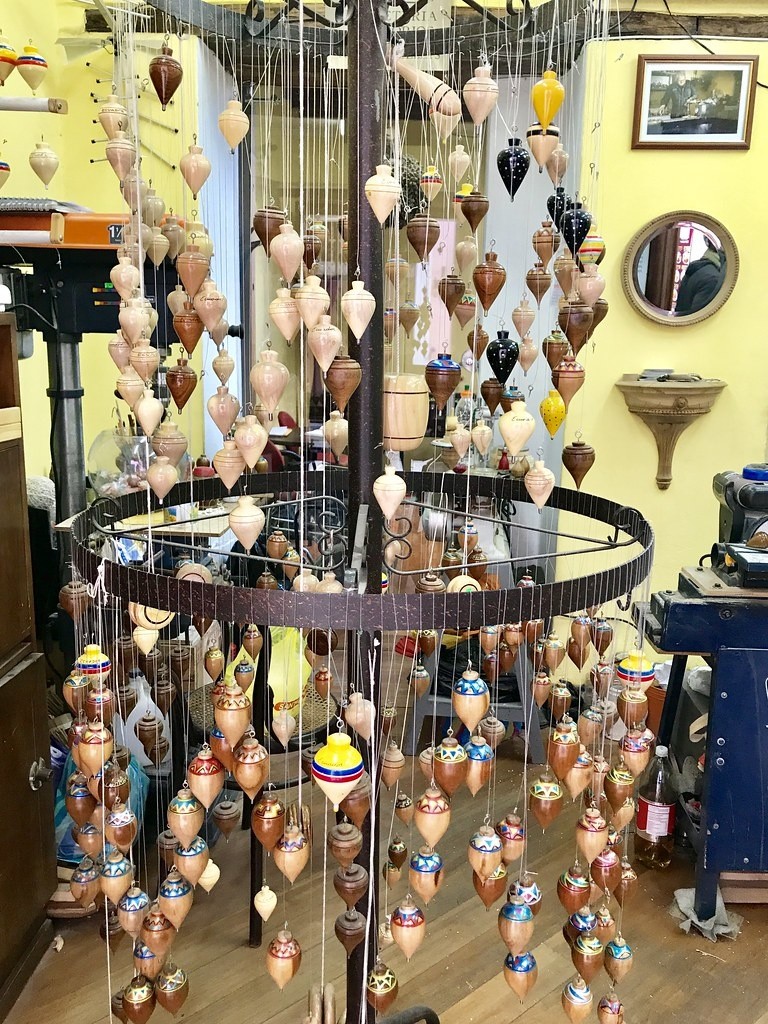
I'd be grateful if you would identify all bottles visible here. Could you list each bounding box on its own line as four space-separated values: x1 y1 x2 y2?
634 745 678 869
115 668 171 767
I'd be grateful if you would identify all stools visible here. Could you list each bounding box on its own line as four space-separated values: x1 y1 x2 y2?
401 557 546 760
183 677 353 948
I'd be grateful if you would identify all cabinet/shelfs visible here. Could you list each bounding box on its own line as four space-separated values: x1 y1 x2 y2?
1 308 61 982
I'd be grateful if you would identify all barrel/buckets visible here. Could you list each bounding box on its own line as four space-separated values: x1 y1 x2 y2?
644 685 667 736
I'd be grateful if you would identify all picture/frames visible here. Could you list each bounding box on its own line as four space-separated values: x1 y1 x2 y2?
632 54 759 150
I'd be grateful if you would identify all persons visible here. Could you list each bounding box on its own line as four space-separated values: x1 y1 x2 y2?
659 73 697 119
675 235 721 312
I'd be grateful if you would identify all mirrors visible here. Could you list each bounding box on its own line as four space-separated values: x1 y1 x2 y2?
622 211 739 328
